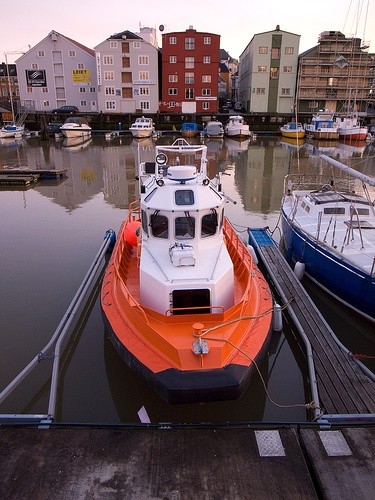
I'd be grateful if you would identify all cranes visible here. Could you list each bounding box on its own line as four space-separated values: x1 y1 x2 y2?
3 44 31 55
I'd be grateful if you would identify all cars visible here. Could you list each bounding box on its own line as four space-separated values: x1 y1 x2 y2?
219 106 229 114
225 102 233 108
51 105 79 116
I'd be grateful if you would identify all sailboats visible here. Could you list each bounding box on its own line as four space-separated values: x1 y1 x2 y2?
0 54 25 138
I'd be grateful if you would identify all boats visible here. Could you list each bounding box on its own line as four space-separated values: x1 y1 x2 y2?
224 134 252 158
279 173 374 326
129 116 155 138
280 121 305 139
99 137 273 405
59 117 92 138
304 109 340 139
335 117 368 141
204 121 224 137
180 123 199 137
62 135 92 152
224 115 252 139
280 136 305 148
